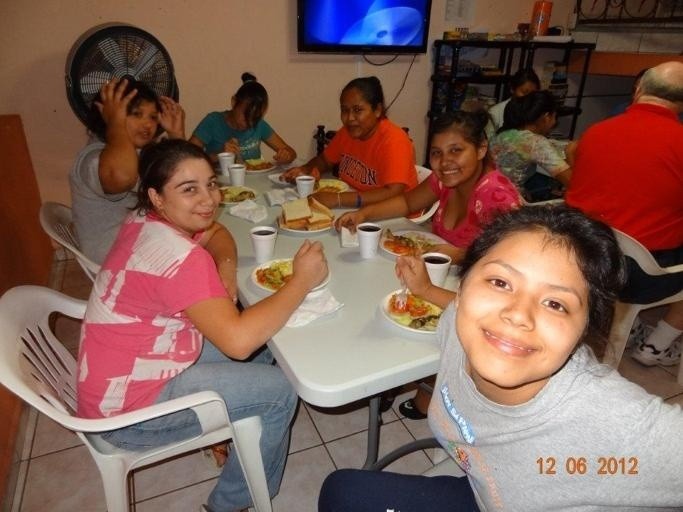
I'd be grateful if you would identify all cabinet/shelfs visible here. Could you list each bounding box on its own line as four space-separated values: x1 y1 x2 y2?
426 40 596 170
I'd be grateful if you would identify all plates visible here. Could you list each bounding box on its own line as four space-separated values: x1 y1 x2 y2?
267 173 289 185
379 231 449 257
249 258 328 292
244 159 276 174
291 178 348 196
219 186 261 205
380 289 443 335
282 226 332 233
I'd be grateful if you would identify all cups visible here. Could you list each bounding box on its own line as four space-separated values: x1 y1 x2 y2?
295 175 313 200
355 222 382 259
249 225 277 262
217 151 235 177
421 253 451 287
226 164 245 187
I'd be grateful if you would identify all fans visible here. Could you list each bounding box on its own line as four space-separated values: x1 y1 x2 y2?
66 22 180 141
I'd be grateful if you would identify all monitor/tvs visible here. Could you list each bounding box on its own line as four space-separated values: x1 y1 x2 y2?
296 1 432 56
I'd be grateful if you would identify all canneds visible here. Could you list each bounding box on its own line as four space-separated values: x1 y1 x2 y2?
530 0 553 37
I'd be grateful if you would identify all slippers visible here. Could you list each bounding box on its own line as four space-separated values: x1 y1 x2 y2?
200 444 249 512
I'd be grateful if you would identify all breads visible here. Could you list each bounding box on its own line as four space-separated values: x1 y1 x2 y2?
276 197 335 231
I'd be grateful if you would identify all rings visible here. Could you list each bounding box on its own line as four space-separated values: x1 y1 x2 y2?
232 296 239 302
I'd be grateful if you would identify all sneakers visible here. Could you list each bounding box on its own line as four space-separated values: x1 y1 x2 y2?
625 317 682 366
399 398 428 421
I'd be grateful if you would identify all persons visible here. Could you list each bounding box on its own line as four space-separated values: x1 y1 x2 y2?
74 139 329 510
278 74 417 211
332 109 531 419
317 201 681 511
564 60 683 370
604 68 650 121
188 69 296 169
64 73 185 270
485 66 541 145
485 90 574 203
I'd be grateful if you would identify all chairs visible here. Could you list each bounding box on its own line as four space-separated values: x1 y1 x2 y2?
39 201 101 284
597 226 683 384
519 193 565 208
408 164 442 225
0 285 273 512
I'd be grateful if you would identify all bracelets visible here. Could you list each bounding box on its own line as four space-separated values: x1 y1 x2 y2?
337 191 343 209
355 192 363 208
